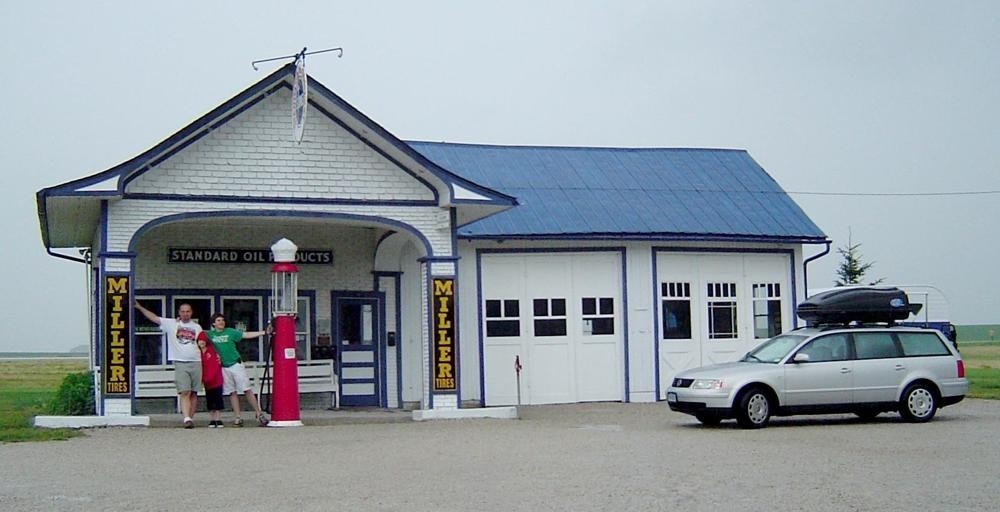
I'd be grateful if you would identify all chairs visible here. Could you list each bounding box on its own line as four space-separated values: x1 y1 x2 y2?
810 347 831 361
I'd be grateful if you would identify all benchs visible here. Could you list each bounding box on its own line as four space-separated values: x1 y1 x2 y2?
94 359 340 414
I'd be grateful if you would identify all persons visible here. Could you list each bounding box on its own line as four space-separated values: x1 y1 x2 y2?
202 313 268 426
195 332 226 428
134 299 204 429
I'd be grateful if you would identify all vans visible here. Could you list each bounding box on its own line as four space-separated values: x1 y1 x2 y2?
666 285 969 427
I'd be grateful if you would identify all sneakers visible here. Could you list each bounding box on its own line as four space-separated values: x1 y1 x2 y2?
183 419 194 428
256 413 268 424
232 419 240 427
217 421 223 427
208 421 216 427
